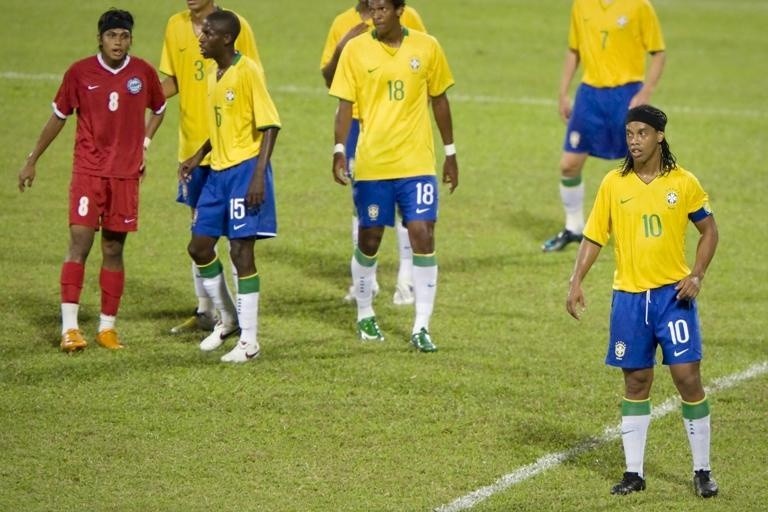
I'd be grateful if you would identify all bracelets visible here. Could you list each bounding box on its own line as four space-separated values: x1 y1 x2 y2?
442 143 455 157
332 143 344 156
142 136 151 149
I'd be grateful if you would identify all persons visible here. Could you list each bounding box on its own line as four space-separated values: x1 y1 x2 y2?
17 10 166 354
157 0 261 336
177 11 281 363
540 0 667 252
328 0 459 354
319 0 428 305
566 104 720 498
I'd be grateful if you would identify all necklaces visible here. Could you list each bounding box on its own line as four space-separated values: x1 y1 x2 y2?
374 32 404 56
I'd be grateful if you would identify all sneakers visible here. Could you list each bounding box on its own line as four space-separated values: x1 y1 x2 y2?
220 338 259 362
541 226 583 252
347 280 415 304
611 471 646 494
693 469 718 497
411 326 436 352
96 327 121 350
357 314 384 341
170 306 219 334
60 328 86 351
199 318 240 352
